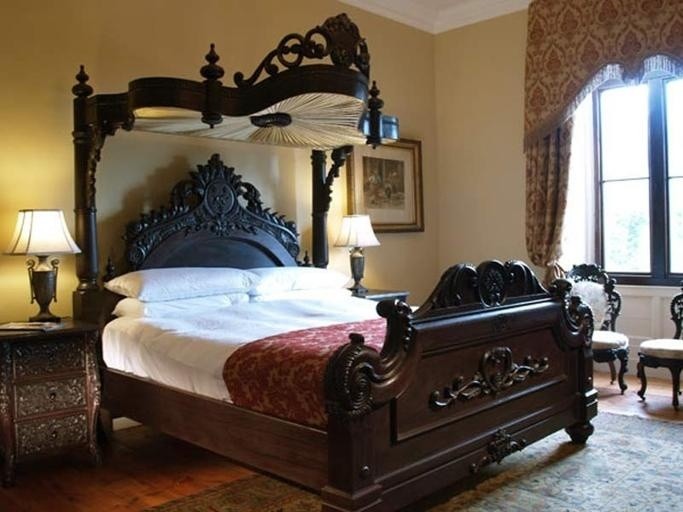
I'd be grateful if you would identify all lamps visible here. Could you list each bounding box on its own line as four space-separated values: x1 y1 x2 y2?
333 215 381 297
0 210 79 322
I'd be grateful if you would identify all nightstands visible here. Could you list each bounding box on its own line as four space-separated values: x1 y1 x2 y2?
0 316 103 486
355 288 409 308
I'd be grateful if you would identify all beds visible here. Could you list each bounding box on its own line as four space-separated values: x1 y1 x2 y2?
73 12 599 506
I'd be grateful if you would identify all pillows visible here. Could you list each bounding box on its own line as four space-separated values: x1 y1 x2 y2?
251 289 353 305
103 268 258 303
111 294 253 318
250 266 351 295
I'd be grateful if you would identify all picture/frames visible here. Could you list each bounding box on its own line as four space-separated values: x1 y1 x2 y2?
346 137 425 234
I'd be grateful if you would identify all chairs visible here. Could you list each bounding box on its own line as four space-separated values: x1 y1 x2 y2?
637 289 682 409
552 263 629 396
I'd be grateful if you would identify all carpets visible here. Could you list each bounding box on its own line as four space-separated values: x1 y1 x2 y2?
130 406 683 509
